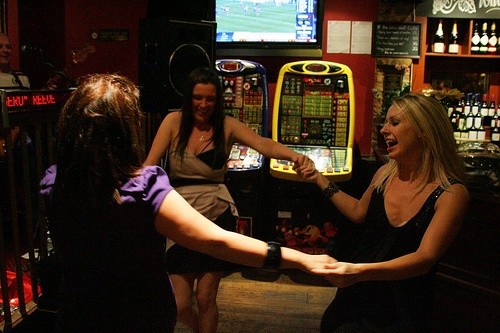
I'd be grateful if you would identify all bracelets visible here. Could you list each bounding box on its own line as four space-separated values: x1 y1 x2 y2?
322 183 339 198
262 241 282 272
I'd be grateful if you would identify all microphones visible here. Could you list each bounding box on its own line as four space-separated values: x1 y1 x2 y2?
20 45 42 53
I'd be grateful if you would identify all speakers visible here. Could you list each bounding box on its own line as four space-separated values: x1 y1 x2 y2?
138 19 215 114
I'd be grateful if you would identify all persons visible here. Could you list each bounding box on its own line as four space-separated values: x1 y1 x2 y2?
0 33 57 158
292 94 472 333
39 72 339 333
142 71 315 333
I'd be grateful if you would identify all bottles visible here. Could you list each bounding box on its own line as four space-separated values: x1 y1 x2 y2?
431 92 500 153
479 20 488 54
497 33 500 55
487 21 497 55
433 19 445 53
471 22 480 54
447 20 459 54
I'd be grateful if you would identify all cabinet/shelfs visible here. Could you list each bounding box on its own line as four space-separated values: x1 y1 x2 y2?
413 16 500 162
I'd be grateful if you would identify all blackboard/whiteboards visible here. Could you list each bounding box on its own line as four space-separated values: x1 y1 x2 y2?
373 22 421 58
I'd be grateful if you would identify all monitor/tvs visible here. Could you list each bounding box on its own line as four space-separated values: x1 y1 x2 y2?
210 0 324 58
